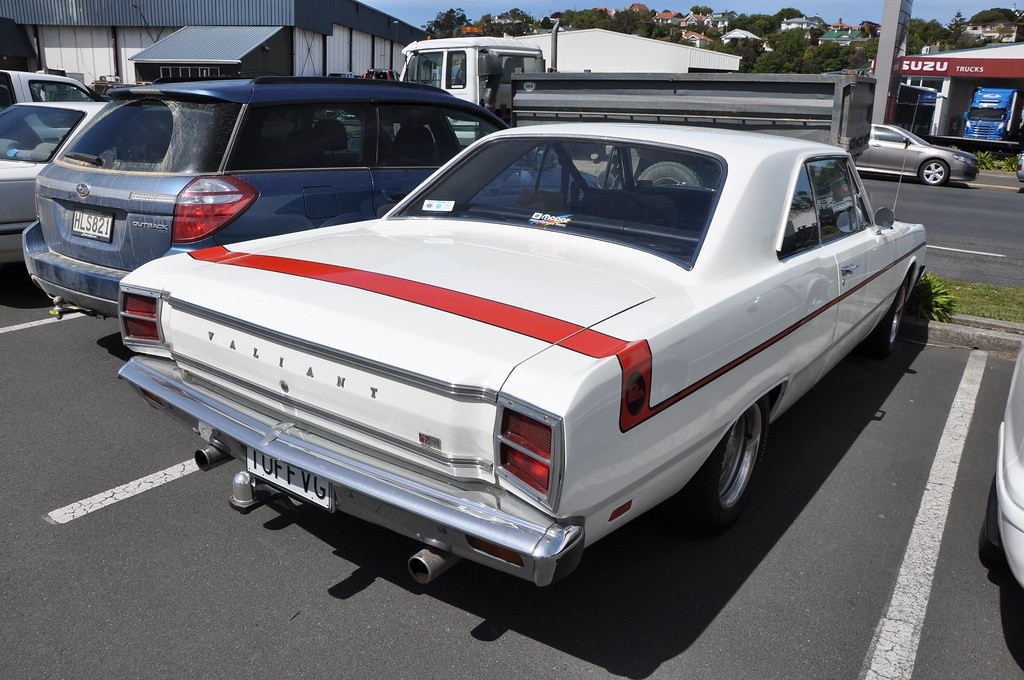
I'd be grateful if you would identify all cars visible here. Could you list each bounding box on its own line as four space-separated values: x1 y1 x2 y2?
4 100 122 262
856 124 977 186
23 76 510 319
4 70 94 102
119 128 925 590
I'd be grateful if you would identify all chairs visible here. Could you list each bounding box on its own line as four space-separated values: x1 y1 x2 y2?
392 109 434 166
501 58 516 84
455 58 465 87
238 114 275 168
312 118 348 167
284 128 322 166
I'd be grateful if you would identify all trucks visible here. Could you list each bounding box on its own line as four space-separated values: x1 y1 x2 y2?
402 41 876 160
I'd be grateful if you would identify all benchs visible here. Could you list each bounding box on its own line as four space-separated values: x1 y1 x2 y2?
573 186 715 254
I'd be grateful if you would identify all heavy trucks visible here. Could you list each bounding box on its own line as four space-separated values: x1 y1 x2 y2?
966 86 1018 144
